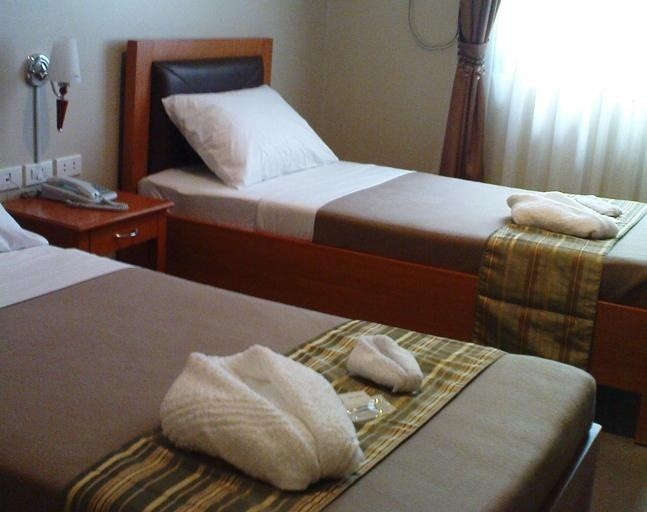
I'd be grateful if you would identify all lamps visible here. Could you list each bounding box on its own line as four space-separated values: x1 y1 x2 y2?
24 33 82 163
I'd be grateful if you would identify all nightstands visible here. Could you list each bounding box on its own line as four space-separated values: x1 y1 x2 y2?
5 191 176 273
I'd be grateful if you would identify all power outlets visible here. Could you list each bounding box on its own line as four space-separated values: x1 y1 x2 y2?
54 155 82 176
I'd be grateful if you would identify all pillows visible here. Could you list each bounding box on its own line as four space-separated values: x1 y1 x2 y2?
0 203 49 252
161 84 339 191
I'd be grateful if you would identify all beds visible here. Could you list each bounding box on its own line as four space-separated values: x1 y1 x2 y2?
116 38 647 446
0 245 602 512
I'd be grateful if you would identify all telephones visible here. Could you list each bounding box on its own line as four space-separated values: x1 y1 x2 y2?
40 175 117 207
